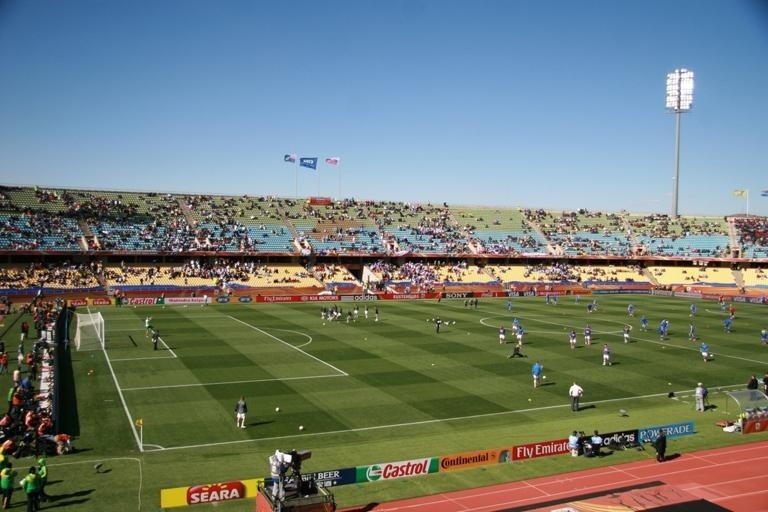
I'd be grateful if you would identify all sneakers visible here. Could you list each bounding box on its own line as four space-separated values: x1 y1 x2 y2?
271 495 276 501
236 424 239 427
241 426 246 428
280 496 285 501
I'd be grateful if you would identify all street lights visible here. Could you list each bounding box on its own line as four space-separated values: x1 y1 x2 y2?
663 66 694 218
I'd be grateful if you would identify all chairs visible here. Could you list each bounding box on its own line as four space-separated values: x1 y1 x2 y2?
314 199 556 288
2 187 314 287
555 210 768 287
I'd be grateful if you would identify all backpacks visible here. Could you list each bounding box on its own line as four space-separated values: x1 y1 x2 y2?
271 461 282 479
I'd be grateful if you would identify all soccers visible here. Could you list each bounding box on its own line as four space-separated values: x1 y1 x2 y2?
299 425 304 430
543 376 546 379
668 383 672 386
276 407 280 412
710 354 714 357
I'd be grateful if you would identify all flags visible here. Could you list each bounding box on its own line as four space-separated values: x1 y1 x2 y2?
284 154 296 163
325 157 339 166
300 157 316 169
